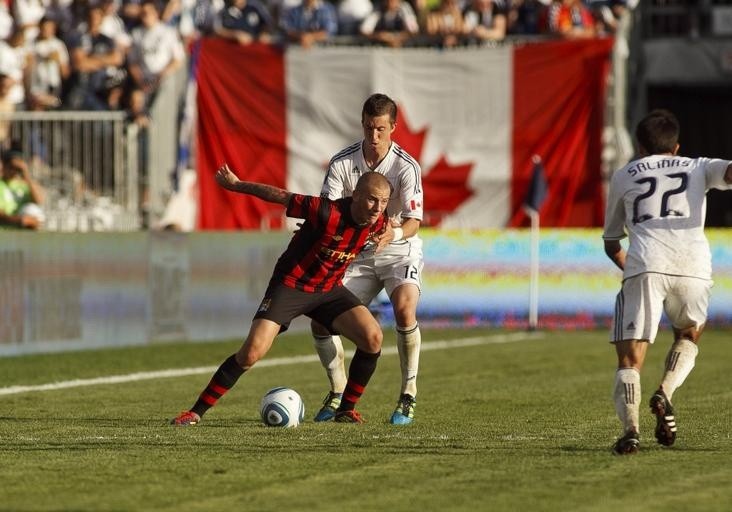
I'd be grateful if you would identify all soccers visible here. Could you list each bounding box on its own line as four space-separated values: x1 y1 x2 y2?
259 386 305 428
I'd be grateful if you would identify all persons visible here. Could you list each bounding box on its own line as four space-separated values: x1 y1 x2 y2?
0 0 629 234
171 159 397 426
599 106 732 454
308 94 425 427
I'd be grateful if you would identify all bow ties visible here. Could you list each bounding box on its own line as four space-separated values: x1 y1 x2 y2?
335 410 367 423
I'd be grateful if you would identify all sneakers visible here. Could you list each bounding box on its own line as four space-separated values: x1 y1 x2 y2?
170 409 200 425
391 387 419 428
610 433 642 457
647 389 679 448
313 388 340 421
